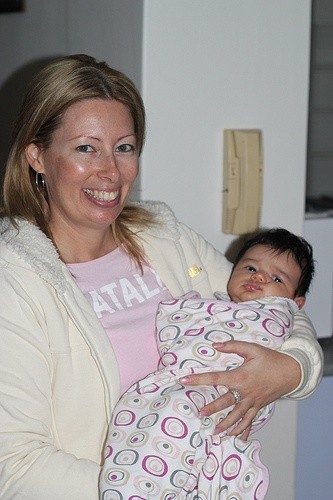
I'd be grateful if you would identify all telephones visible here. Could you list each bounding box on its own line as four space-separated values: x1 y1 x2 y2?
221 127 264 237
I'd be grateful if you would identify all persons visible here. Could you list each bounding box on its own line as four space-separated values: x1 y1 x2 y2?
98 226 318 500
0 51 325 500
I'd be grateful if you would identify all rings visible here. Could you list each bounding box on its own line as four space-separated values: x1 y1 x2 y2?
228 387 241 406
